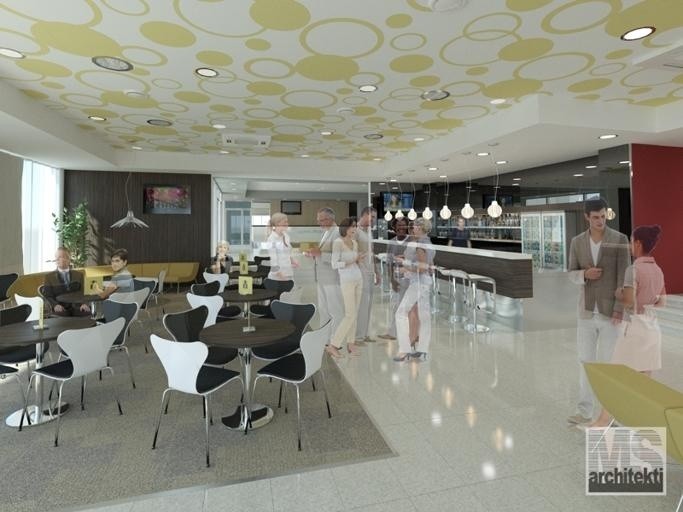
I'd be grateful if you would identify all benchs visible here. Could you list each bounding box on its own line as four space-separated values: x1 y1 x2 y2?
584 363 683 512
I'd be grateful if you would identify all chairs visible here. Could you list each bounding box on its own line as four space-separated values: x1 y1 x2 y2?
0 255 333 467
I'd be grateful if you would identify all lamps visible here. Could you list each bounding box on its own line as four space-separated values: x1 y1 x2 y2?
110 147 149 229
383 146 502 222
606 172 616 220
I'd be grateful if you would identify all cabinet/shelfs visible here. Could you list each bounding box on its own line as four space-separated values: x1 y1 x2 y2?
385 207 521 242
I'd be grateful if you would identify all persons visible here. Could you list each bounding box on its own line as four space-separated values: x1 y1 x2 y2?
266 203 436 362
565 195 633 423
93 247 135 302
210 240 233 275
575 226 667 428
448 217 472 248
388 194 402 208
42 246 91 313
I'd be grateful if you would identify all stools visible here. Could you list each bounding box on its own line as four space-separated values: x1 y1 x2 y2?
373 253 496 313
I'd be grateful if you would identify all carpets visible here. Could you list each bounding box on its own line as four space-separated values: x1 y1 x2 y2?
0 293 400 511
